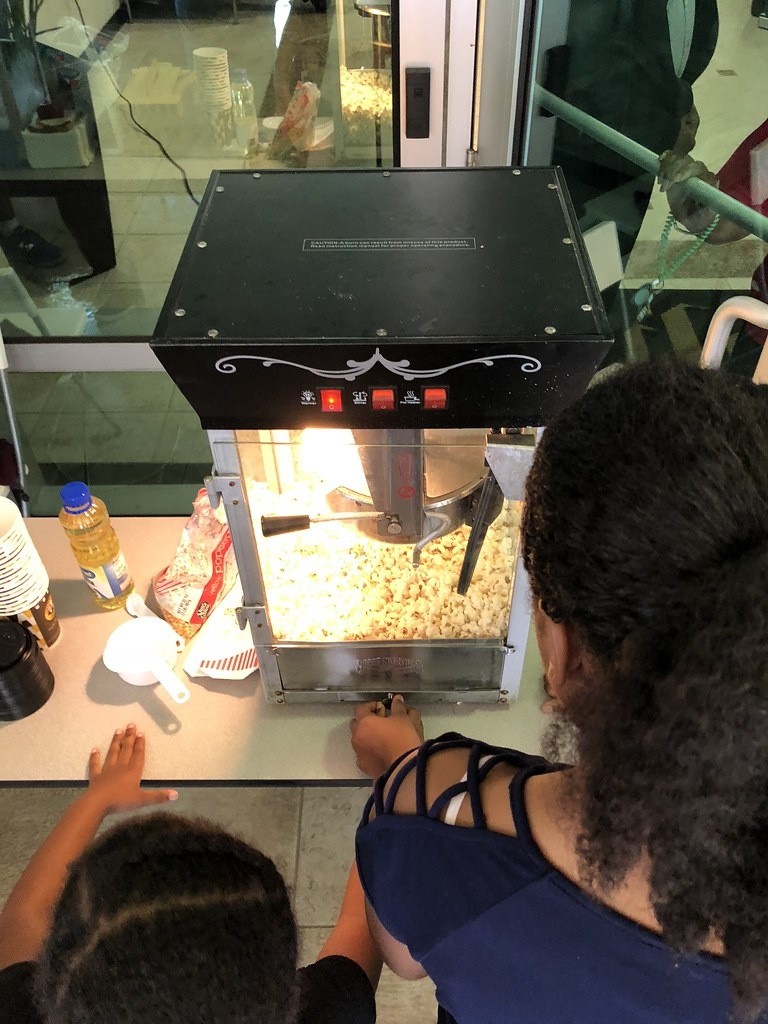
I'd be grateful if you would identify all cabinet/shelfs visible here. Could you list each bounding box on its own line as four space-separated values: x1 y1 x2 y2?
0 42 116 295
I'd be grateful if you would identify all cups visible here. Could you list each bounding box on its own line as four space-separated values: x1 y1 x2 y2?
193 47 233 150
0 494 61 651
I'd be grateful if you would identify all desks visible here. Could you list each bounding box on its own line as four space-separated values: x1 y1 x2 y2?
0 515 556 787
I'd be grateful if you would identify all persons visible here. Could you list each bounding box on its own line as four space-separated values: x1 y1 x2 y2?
659 117 768 386
0 362 768 1024
551 1 720 273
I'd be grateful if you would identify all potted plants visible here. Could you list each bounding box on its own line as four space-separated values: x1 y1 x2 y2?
0 0 59 142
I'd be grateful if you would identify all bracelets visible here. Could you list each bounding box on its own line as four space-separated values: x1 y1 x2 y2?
673 205 725 236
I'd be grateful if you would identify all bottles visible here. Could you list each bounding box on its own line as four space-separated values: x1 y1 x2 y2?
231 68 258 147
59 481 135 611
